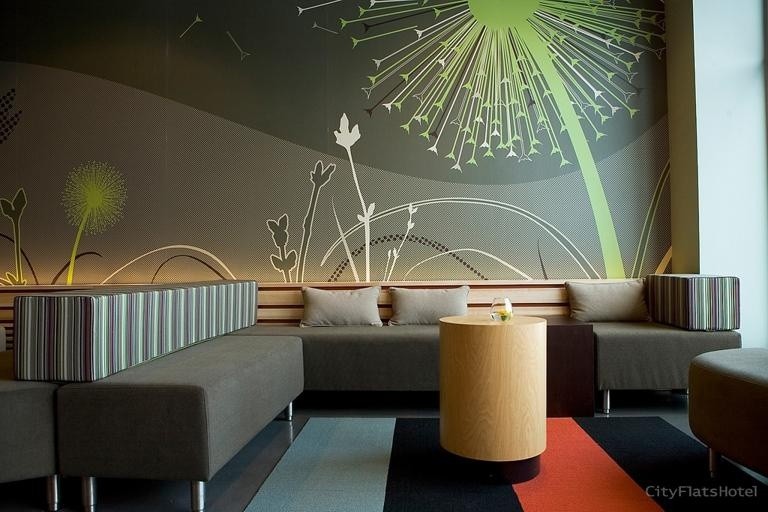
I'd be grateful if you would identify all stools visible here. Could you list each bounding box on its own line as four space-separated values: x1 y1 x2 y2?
688 348 768 481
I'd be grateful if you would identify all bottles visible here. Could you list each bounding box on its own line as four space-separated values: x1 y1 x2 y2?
491 296 514 321
